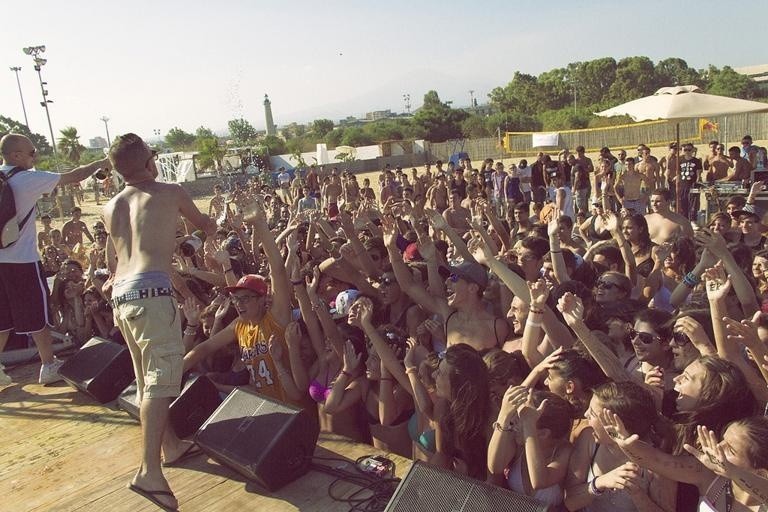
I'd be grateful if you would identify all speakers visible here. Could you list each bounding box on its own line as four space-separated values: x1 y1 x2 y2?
193 386 320 492
384 459 552 512
119 366 221 431
58 335 135 403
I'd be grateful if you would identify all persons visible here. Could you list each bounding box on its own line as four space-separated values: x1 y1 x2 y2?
100 133 219 510
33 172 127 346
0 133 112 387
177 135 768 512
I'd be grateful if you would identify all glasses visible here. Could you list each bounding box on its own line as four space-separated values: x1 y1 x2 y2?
684 148 693 152
629 328 664 343
449 273 460 282
669 332 690 345
17 148 37 156
741 142 750 145
144 153 158 169
595 281 622 294
716 148 723 151
230 296 263 304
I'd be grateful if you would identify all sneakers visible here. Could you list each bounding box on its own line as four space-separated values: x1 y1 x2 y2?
0 369 12 386
39 359 65 385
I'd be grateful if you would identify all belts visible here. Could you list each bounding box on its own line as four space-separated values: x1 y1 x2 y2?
110 287 177 310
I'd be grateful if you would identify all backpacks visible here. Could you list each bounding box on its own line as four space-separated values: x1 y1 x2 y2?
0 166 35 249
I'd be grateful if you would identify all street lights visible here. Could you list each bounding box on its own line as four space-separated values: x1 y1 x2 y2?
467 89 474 108
22 44 63 174
9 66 30 132
444 100 452 108
99 114 110 150
569 77 581 117
401 93 412 118
152 127 163 155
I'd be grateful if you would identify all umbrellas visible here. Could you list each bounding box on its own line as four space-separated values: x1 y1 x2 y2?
592 84 768 216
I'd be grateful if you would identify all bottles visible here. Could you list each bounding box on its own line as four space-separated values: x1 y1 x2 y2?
93 153 113 180
180 228 209 255
740 176 745 189
754 148 765 170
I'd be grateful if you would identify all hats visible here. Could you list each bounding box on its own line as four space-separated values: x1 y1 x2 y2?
331 290 358 320
670 142 678 149
225 275 268 296
438 262 487 289
731 205 764 220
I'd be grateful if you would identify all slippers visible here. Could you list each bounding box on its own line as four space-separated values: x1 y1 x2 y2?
163 439 200 468
129 482 179 512
